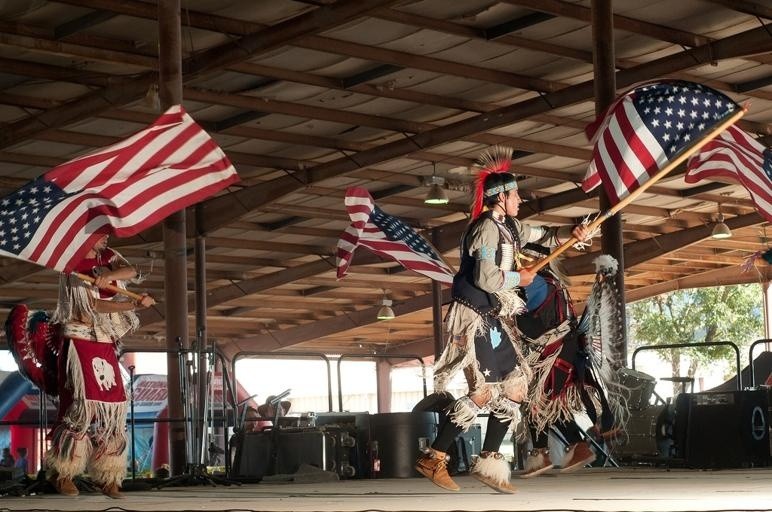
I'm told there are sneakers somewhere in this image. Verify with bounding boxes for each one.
[47,474,82,497]
[555,441,599,473]
[517,447,554,479]
[413,448,460,493]
[104,478,128,501]
[467,458,518,496]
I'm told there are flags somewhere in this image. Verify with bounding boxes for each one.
[683,119,771,225]
[0,99,242,276]
[332,182,456,287]
[580,75,743,209]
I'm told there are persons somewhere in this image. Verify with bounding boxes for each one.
[42,228,157,500]
[413,168,591,495]
[511,240,597,479]
[1,447,15,468]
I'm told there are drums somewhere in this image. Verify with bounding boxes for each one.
[617,369,654,411]
[619,404,669,461]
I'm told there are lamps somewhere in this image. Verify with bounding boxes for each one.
[712,201,733,239]
[376,288,395,319]
[424,161,449,204]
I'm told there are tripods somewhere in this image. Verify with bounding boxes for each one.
[151,354,243,488]
[20,387,56,494]
